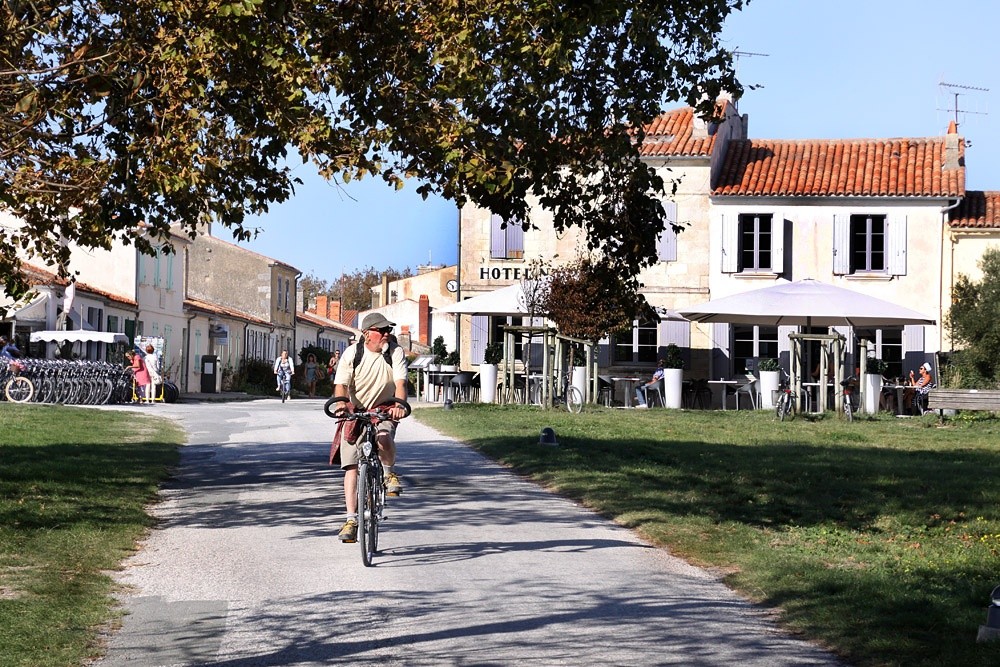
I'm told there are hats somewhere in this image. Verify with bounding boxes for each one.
[923,362,932,371]
[361,313,397,329]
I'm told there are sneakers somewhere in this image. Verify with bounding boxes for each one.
[337,520,359,540]
[381,472,403,493]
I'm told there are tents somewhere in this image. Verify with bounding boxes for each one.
[30,330,129,360]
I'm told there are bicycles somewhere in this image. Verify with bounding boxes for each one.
[536,371,583,414]
[324,396,411,567]
[276,371,291,403]
[775,368,799,422]
[0,358,179,405]
[840,375,856,423]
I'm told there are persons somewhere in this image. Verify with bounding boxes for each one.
[274,350,294,400]
[304,353,319,398]
[902,362,933,415]
[635,361,665,408]
[142,344,159,404]
[333,313,407,540]
[0,335,20,358]
[329,350,341,397]
[812,362,821,411]
[125,350,151,404]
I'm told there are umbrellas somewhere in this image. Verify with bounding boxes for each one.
[430,274,691,405]
[674,274,936,415]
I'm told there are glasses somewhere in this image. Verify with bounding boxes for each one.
[365,326,393,335]
[335,353,340,355]
[919,367,924,370]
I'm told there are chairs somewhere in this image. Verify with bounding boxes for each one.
[590,374,664,410]
[689,379,756,410]
[425,370,480,404]
[496,371,560,404]
[791,380,930,416]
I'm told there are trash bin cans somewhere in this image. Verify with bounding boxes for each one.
[201,355,220,392]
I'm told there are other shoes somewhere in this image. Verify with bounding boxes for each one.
[276,387,280,391]
[635,404,646,408]
[287,396,290,401]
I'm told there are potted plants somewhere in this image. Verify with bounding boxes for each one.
[865,355,888,415]
[567,348,586,404]
[441,351,459,402]
[427,335,447,402]
[479,342,503,403]
[664,342,686,410]
[758,358,780,410]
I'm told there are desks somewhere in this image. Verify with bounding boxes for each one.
[882,383,919,417]
[802,383,834,411]
[610,378,642,408]
[708,380,737,411]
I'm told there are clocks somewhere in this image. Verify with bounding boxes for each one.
[446,280,457,292]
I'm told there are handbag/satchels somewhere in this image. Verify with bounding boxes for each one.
[327,366,333,374]
[314,370,322,378]
